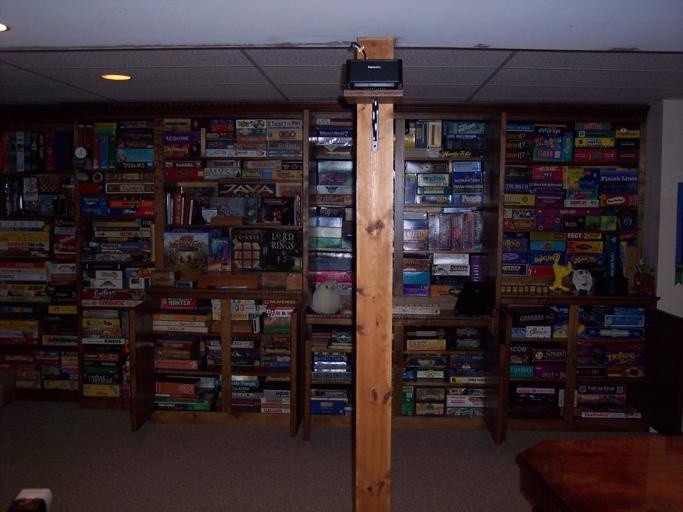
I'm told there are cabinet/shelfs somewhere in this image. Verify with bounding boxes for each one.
[0,112,646,439]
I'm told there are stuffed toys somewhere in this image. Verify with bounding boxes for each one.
[548,253,574,291]
[447,278,484,316]
[310,279,342,315]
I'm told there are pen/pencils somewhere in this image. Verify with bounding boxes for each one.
[632,257,655,276]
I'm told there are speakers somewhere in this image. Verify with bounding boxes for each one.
[346,56,404,90]
